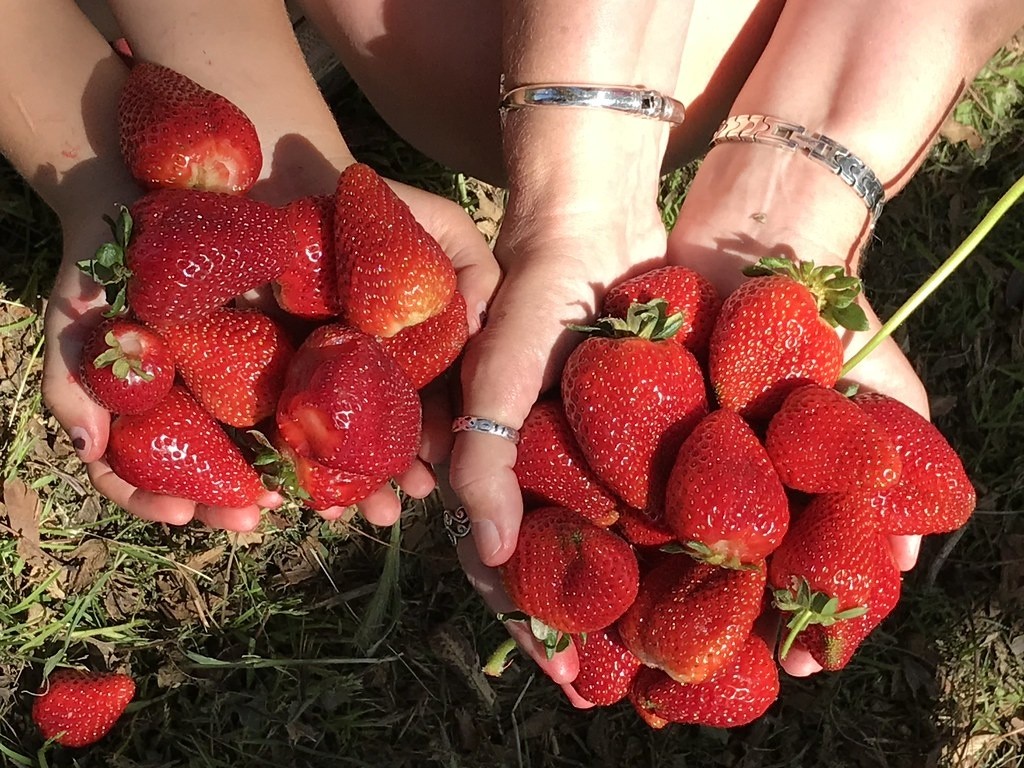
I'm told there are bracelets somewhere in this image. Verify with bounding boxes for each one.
[707,115,885,221]
[498,83,686,129]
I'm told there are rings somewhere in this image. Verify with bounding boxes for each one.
[443,505,472,546]
[452,416,520,445]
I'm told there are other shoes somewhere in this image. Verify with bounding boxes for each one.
[283,0,340,83]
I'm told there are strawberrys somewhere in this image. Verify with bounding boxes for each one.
[116,61,263,195]
[77,162,469,512]
[500,257,977,730]
[32,669,136,747]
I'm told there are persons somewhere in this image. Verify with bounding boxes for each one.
[297,0,1024,730]
[0,0,503,533]
